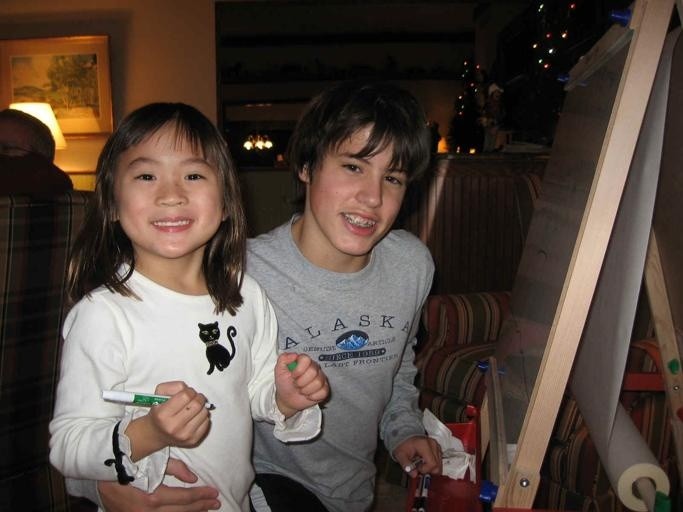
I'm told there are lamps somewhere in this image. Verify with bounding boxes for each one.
[243,134,273,150]
[8,102,66,151]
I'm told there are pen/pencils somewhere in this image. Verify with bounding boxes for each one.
[406,460,425,472]
[412,473,430,512]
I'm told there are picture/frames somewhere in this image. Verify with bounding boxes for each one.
[0,35,114,136]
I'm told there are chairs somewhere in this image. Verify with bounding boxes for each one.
[384,169,682,511]
[0,190,99,511]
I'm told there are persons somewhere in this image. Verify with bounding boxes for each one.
[62,86,443,512]
[46,102,330,511]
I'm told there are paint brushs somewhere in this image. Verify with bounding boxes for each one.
[99,388,216,414]
